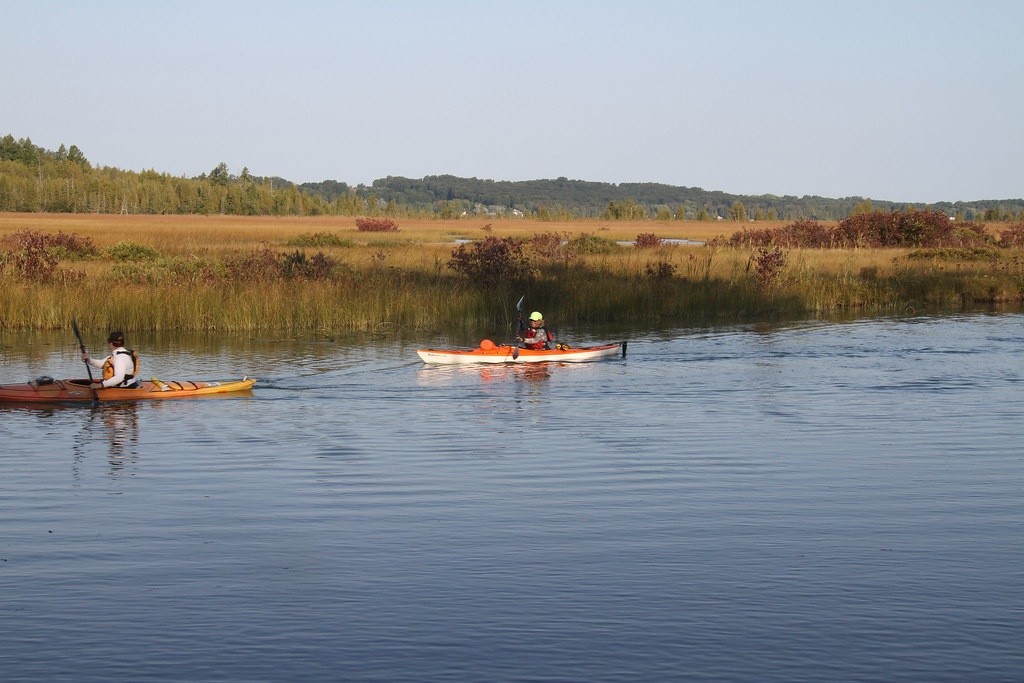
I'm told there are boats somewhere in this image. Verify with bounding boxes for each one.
[416,341,627,364]
[0,375,256,402]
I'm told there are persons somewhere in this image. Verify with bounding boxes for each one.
[515,312,548,351]
[81,331,139,389]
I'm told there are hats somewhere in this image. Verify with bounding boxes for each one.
[106,332,125,342]
[529,311,543,321]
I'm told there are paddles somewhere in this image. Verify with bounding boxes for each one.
[71,317,103,407]
[512,295,525,360]
